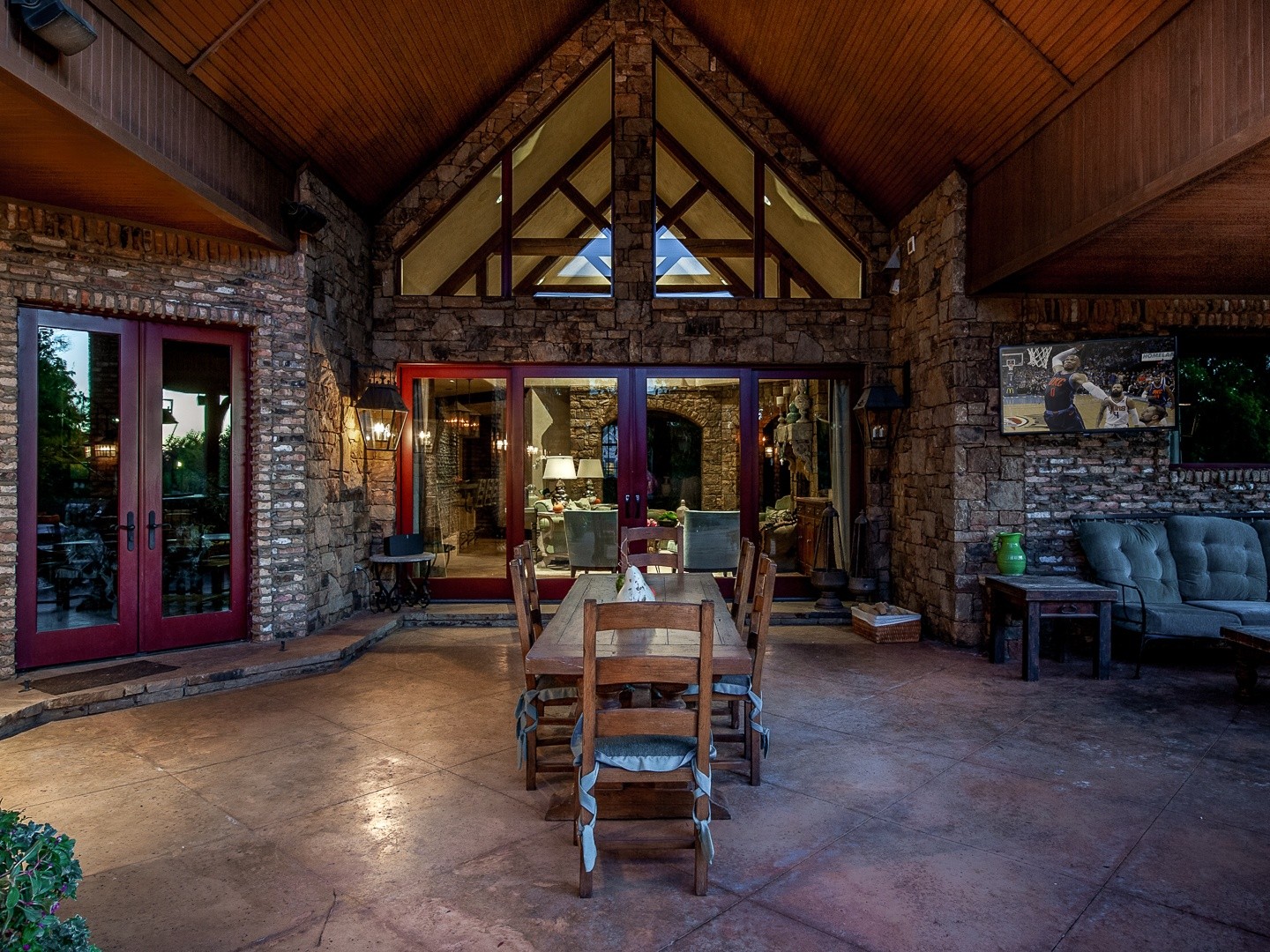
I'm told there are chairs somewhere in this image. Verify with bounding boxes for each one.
[758,492,794,573]
[507,560,582,792]
[619,524,684,573]
[515,541,548,640]
[649,536,754,727]
[562,509,617,577]
[571,596,719,897]
[534,500,571,567]
[682,509,740,577]
[684,553,778,787]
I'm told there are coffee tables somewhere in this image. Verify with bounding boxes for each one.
[1219,624,1270,719]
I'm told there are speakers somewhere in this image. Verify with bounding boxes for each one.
[384,533,423,556]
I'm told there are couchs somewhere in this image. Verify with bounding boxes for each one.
[1074,512,1270,680]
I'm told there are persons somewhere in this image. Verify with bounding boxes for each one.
[1141,373,1176,411]
[647,471,659,506]
[1139,404,1167,427]
[1043,346,1118,431]
[1095,383,1140,428]
[1002,361,1175,397]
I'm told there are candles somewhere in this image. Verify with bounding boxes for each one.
[776,396,784,405]
[782,386,791,394]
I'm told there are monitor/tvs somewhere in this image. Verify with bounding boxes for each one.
[999,333,1179,433]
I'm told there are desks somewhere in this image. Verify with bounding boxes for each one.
[525,572,754,822]
[368,551,433,611]
[590,503,618,509]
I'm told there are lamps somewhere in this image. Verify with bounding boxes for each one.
[353,367,412,451]
[576,457,604,504]
[441,378,468,435]
[464,379,485,438]
[413,405,442,455]
[852,363,912,452]
[542,452,577,508]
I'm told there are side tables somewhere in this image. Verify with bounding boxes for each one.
[987,575,1118,681]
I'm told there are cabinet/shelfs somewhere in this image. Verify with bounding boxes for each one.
[796,495,828,576]
